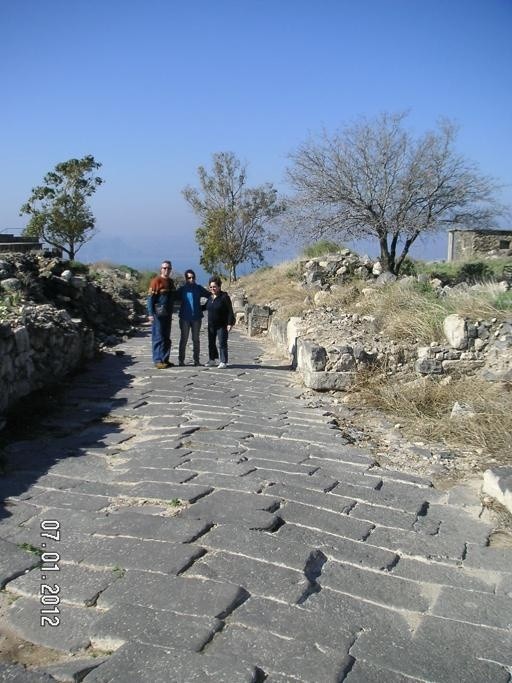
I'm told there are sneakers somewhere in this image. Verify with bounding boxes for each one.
[205,360,215,366]
[179,362,184,365]
[155,362,173,369]
[194,363,203,366]
[218,363,226,368]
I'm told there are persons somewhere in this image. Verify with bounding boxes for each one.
[147,260,176,368]
[200,275,235,369]
[175,268,211,365]
